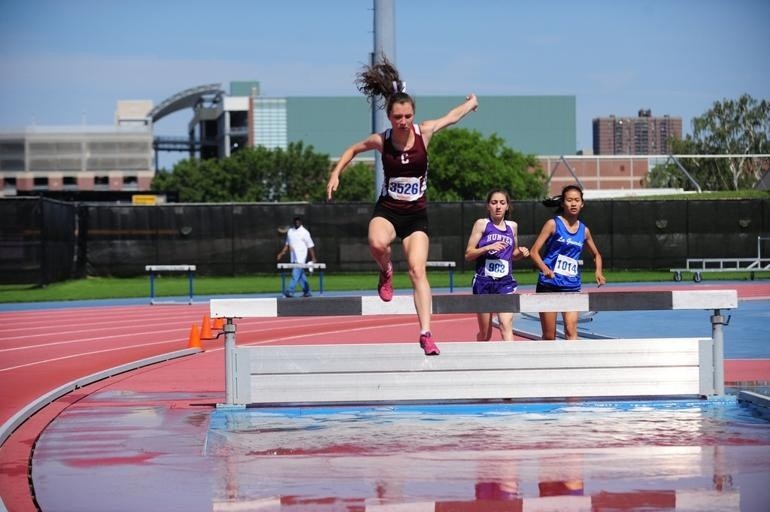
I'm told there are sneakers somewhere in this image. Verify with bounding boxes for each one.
[285,290,312,297]
[378,270,393,301]
[420,332,440,355]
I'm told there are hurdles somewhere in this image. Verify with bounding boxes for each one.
[209,290,738,408]
[425,260,457,292]
[276,262,327,296]
[146,265,195,305]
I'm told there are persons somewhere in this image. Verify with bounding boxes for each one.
[463,190,530,342]
[482,398,585,500]
[530,185,607,340]
[327,48,480,354]
[276,216,317,298]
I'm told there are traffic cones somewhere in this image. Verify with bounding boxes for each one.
[188,313,224,348]
